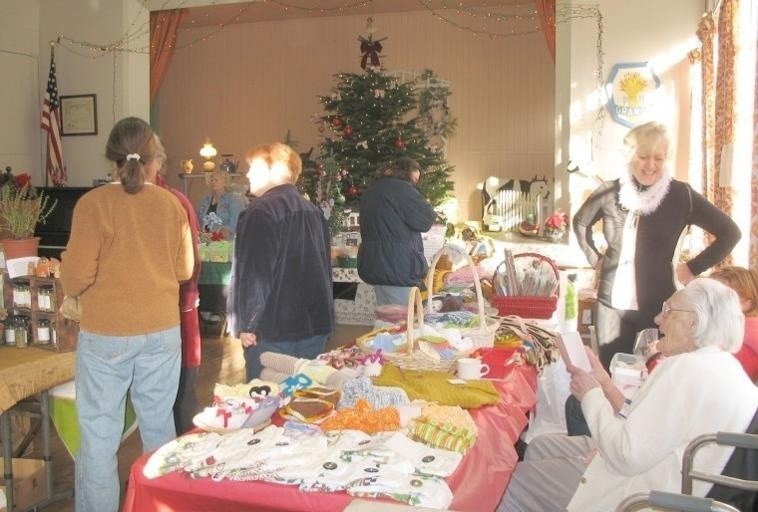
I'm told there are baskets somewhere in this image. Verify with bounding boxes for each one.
[491,252,562,320]
[354,285,456,375]
[425,243,500,350]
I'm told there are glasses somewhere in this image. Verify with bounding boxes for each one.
[661,300,693,316]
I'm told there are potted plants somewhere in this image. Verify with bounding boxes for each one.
[544,210,568,240]
[1,180,58,259]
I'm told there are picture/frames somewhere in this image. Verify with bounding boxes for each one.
[60,94,98,136]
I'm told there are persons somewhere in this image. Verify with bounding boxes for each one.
[56,116,194,512]
[145,132,202,438]
[357,156,437,309]
[571,120,741,378]
[492,276,758,512]
[195,169,247,239]
[564,264,756,437]
[224,143,337,384]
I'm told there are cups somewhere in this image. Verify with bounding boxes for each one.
[457,359,490,381]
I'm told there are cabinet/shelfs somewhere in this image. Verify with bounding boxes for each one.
[0,274,80,352]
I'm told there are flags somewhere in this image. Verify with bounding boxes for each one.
[38,58,69,189]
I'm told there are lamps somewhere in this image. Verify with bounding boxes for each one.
[199,143,217,171]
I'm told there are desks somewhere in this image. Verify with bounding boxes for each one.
[0,344,77,511]
[123,327,537,512]
[33,186,96,249]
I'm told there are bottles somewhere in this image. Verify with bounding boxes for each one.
[37,321,50,343]
[3,322,16,347]
[16,323,28,349]
[38,288,55,312]
[14,284,30,309]
[52,324,58,348]
